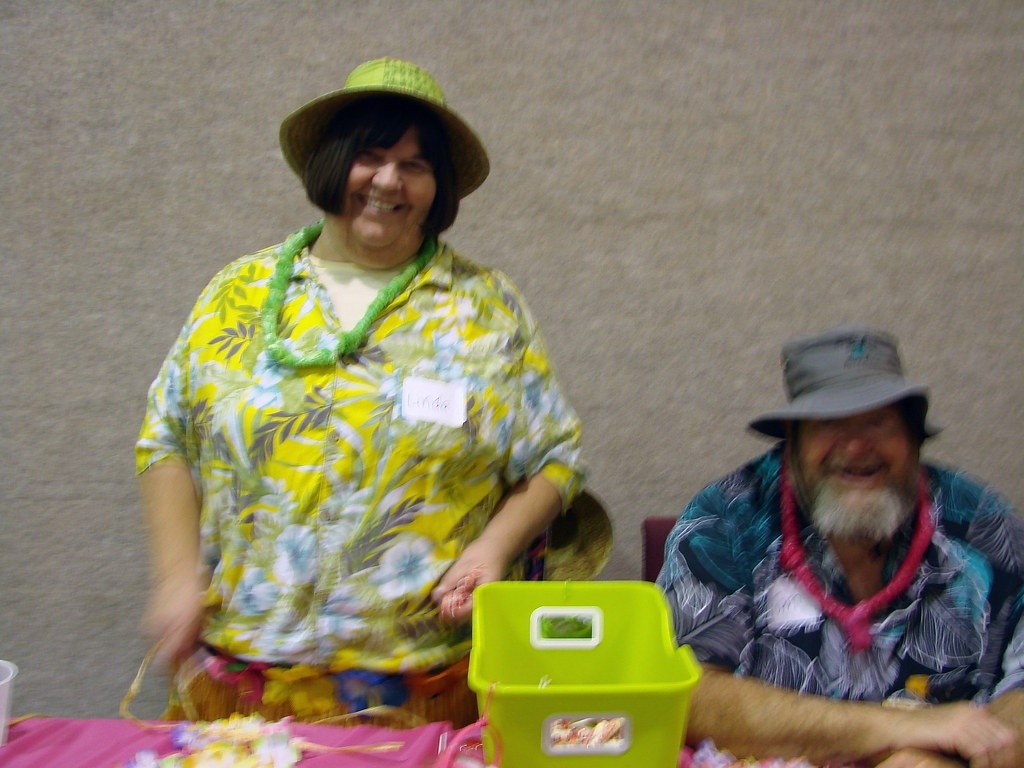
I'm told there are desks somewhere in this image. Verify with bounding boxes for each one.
[1,718,489,768]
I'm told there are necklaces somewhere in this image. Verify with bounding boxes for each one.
[780,462,932,651]
[263,222,437,368]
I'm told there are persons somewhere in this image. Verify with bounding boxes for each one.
[132,58,584,727]
[655,326,1024,768]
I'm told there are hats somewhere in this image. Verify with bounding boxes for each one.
[278,55,491,203]
[745,324,951,443]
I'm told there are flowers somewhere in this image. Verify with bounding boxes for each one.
[695,738,812,768]
[118,712,303,767]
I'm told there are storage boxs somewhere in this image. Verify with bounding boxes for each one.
[467,582,704,768]
